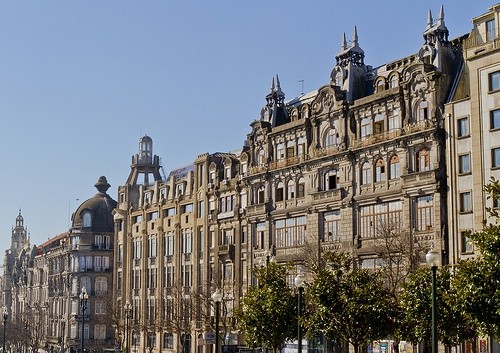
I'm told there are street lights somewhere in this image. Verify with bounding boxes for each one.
[123,300,133,353]
[425,247,441,353]
[2,309,8,353]
[79,286,89,353]
[294,274,306,353]
[212,289,221,353]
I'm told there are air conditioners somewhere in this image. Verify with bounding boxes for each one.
[239,175,243,180]
[208,182,213,189]
[240,208,244,213]
[223,180,228,184]
[207,215,213,219]
